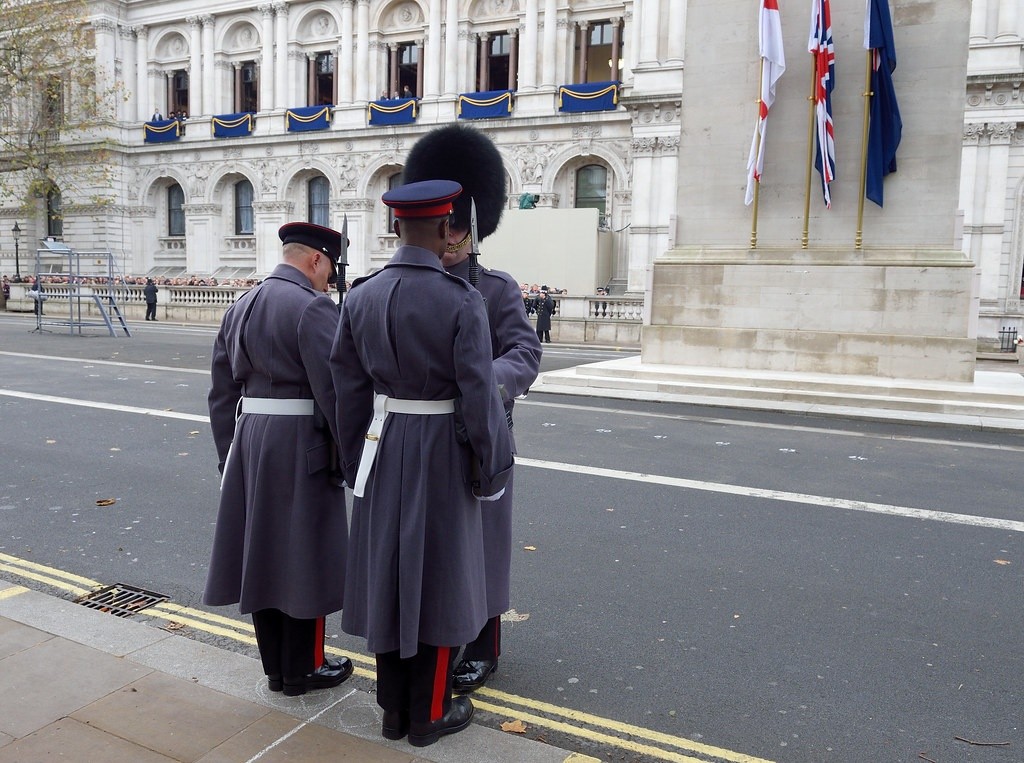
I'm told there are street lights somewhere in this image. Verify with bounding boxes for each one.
[11,220,24,283]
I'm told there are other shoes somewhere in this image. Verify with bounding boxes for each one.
[546,340,550,343]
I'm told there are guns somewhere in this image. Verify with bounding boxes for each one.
[467,197,488,306]
[335,213,349,315]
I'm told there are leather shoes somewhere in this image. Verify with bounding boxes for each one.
[282,656,354,696]
[382,709,408,740]
[451,657,498,691]
[268,674,283,692]
[408,696,475,747]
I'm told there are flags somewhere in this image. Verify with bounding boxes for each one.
[744,1,787,206]
[862,1,904,207]
[807,1,837,208]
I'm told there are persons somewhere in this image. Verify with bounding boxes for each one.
[151,109,189,135]
[380,84,413,101]
[2,273,353,305]
[330,125,542,747]
[203,221,356,696]
[144,279,158,321]
[519,284,610,343]
[32,278,47,316]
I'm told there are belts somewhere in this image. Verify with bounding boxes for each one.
[242,396,314,416]
[353,390,455,498]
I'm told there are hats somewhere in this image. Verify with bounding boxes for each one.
[597,287,604,292]
[382,180,463,220]
[278,222,350,284]
[540,285,548,294]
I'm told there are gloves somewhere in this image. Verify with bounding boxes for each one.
[472,486,506,501]
